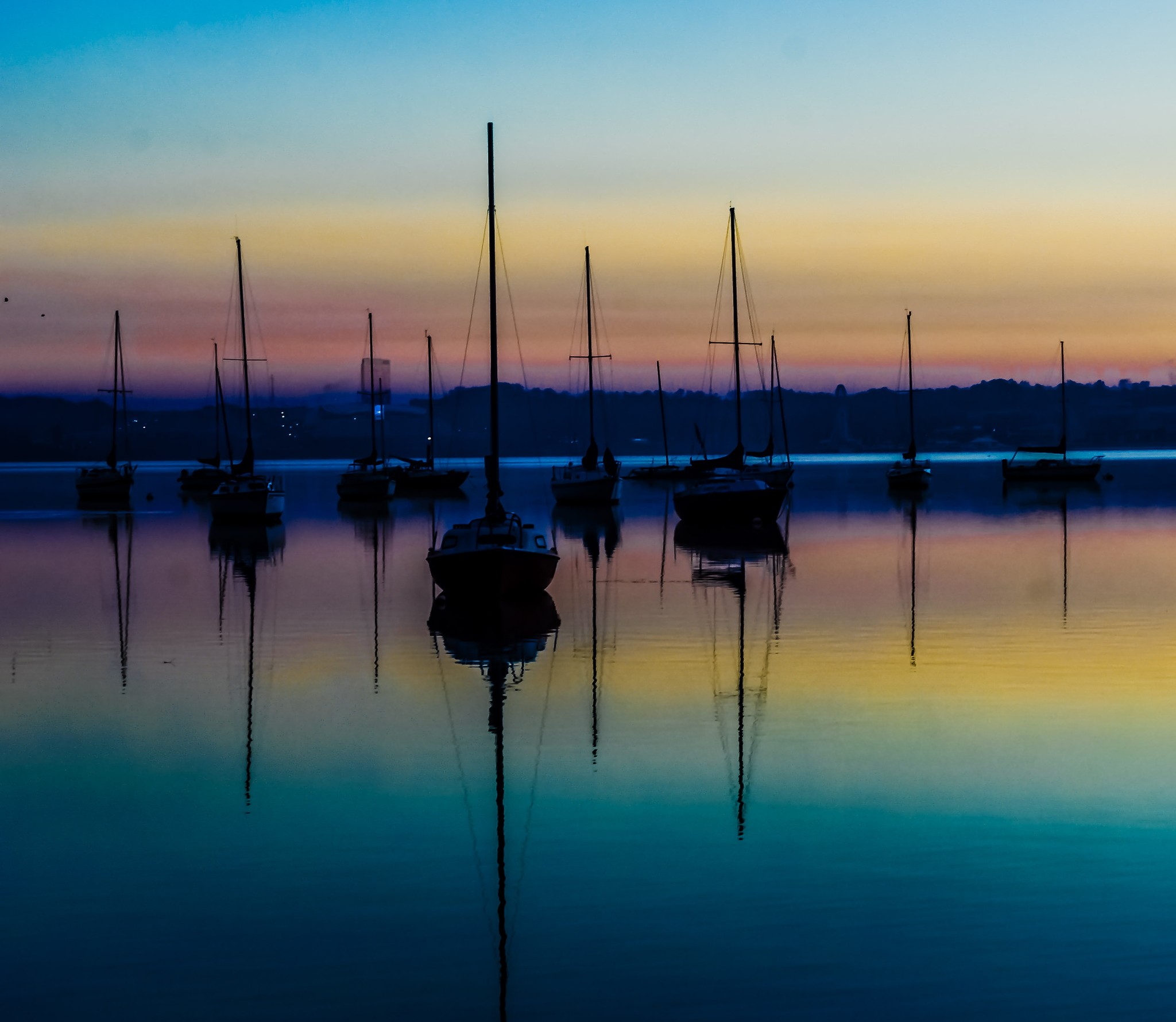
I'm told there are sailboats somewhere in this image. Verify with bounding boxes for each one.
[426,122,562,601]
[73,310,139,510]
[884,309,933,487]
[549,245,624,506]
[177,238,286,523]
[1001,342,1106,480]
[334,312,471,503]
[623,206,798,531]
